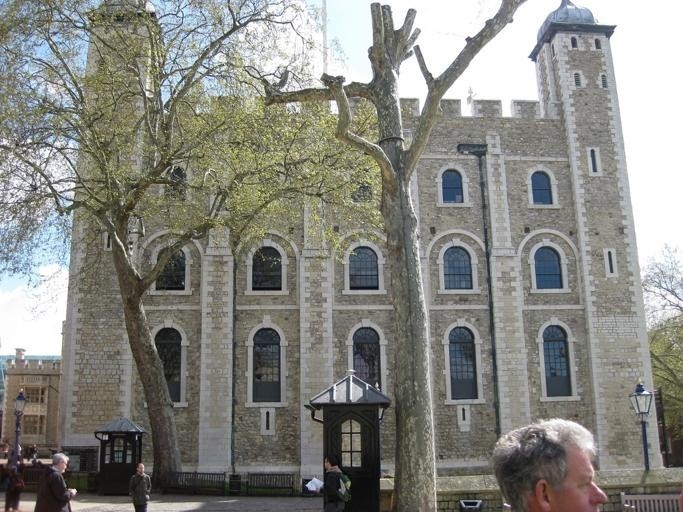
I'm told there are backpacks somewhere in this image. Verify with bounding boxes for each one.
[333,472,352,503]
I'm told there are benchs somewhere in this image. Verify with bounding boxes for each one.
[20,467,45,489]
[161,471,295,497]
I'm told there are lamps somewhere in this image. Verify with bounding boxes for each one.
[101,433,112,442]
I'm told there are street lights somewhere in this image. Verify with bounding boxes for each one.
[629,382,652,471]
[14,388,26,465]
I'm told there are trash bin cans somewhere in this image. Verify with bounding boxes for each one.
[459,499,482,512]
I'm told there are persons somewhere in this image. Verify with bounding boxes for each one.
[128,462,152,512]
[491,417,609,511]
[33,452,77,512]
[17,443,24,465]
[31,443,40,464]
[314,454,351,512]
[2,440,10,459]
[4,465,25,511]
